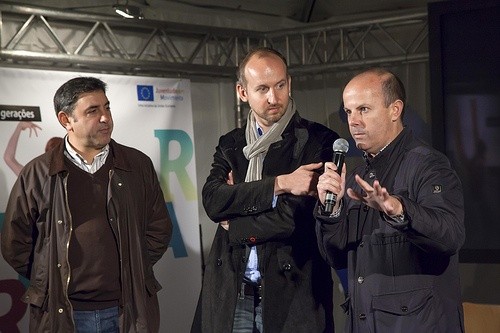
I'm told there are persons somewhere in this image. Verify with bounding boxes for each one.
[188,48,342,333]
[1,77,173,333]
[313,69,467,333]
[4,121,62,178]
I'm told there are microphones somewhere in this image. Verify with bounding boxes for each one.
[324,138,350,214]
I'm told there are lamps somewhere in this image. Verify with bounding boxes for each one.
[112,0,144,19]
